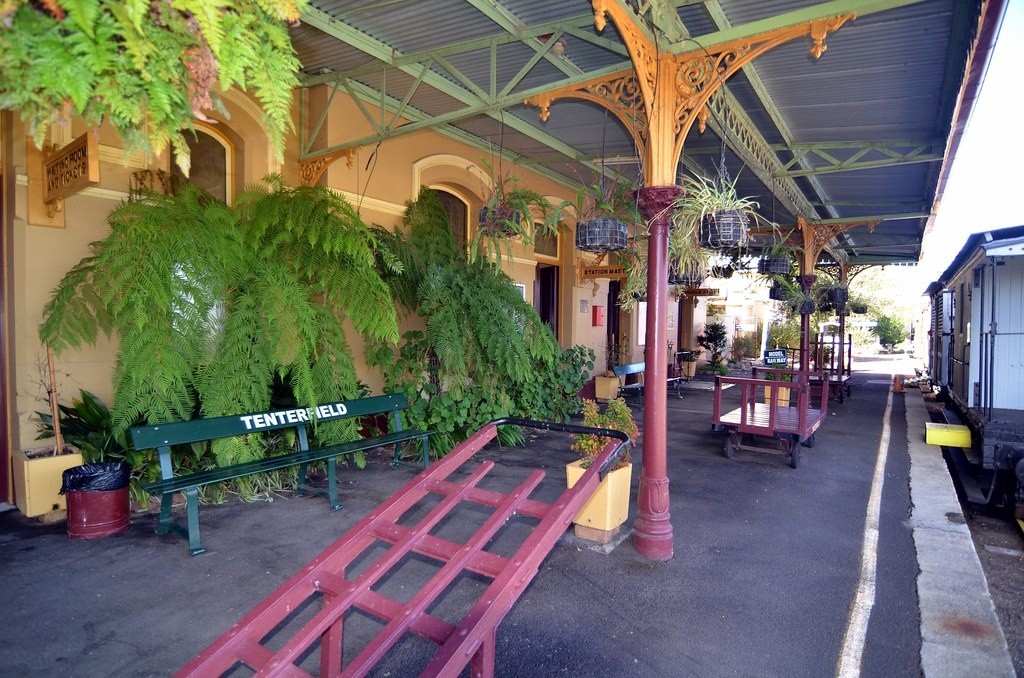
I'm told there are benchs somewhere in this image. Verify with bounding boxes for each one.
[612,362,685,411]
[130,393,438,557]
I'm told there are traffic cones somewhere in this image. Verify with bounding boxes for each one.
[890,376,902,393]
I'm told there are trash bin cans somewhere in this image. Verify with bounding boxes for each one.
[678,351,697,381]
[63,462,131,539]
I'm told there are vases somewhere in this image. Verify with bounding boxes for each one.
[14,442,82,518]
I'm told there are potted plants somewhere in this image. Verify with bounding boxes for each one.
[697,321,728,376]
[811,345,833,369]
[649,163,871,316]
[35,150,650,541]
[765,361,797,407]
[593,335,629,405]
